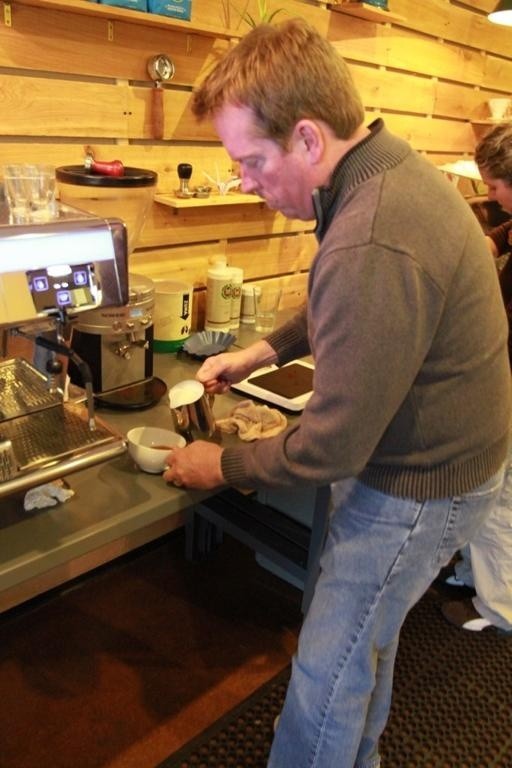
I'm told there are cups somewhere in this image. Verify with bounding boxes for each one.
[2,161,57,219]
[168,380,217,443]
[253,286,283,333]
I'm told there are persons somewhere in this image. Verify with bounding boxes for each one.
[412,116,511,639]
[158,13,512,767]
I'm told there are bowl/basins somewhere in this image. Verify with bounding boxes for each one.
[127,425,187,475]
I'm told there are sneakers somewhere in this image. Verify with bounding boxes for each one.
[441,574,478,635]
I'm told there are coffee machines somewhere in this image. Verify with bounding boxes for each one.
[64,272,167,409]
